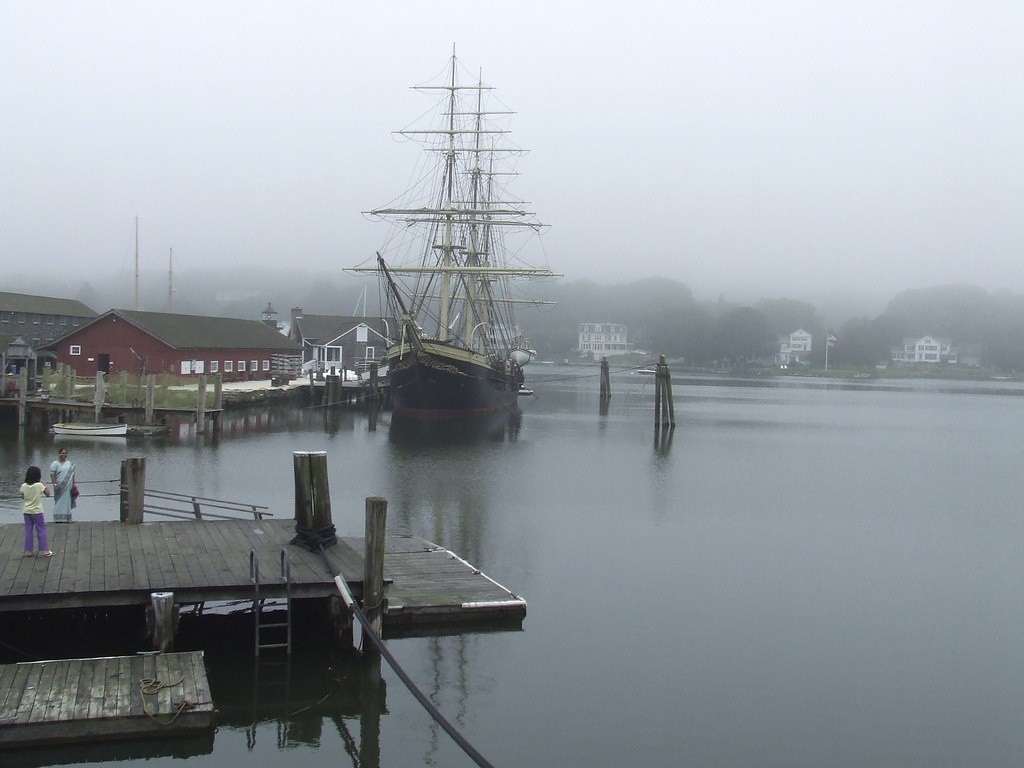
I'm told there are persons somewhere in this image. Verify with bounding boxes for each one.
[50,448,77,523]
[20,467,56,557]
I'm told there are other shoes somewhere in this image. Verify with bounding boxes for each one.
[44,551,56,557]
[23,552,32,557]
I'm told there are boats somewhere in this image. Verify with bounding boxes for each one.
[636,367,656,375]
[52,422,129,436]
[52,434,128,448]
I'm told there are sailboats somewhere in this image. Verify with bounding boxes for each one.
[340,41,564,421]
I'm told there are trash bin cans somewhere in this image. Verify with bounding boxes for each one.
[41,389,50,402]
[272,375,280,387]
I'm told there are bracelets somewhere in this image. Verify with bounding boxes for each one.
[54,484,57,486]
[73,483,77,485]
